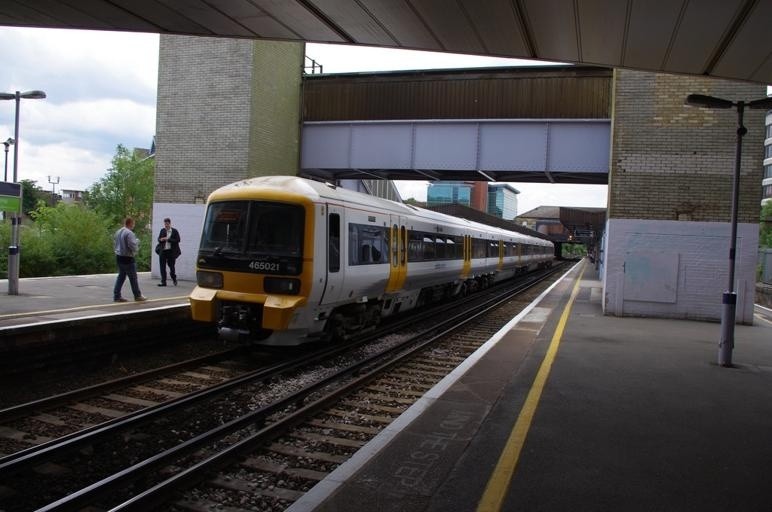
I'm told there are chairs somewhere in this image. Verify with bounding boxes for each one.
[135,296,146,301]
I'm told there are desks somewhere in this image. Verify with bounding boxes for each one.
[684,92,772,369]
[1,137,16,220]
[0,91,46,294]
[47,175,60,206]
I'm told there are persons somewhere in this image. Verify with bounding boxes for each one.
[112,217,148,301]
[155,218,180,287]
